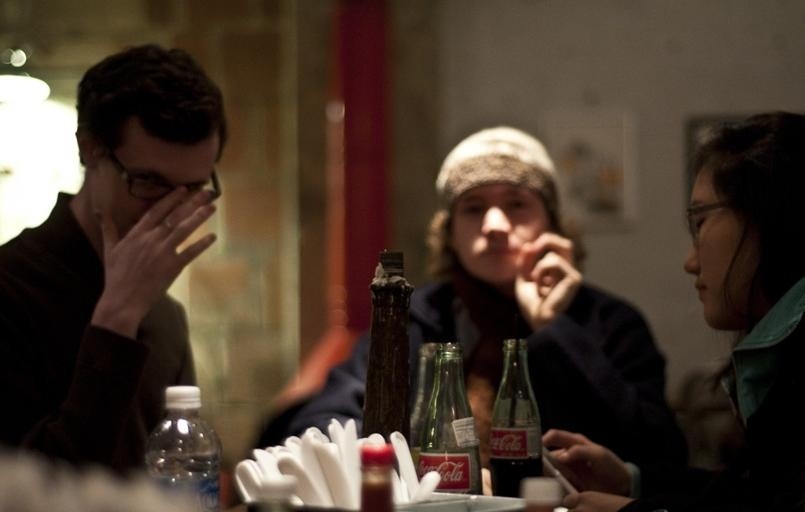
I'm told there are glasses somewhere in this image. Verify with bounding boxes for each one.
[685,200,728,250]
[104,150,221,204]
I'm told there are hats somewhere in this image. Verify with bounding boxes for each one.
[435,125,559,214]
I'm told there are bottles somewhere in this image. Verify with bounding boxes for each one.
[409,343,437,467]
[362,251,415,438]
[144,385,221,512]
[414,342,485,496]
[485,339,543,499]
[359,446,395,512]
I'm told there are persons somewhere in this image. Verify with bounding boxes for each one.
[0,45,231,512]
[291,125,690,465]
[541,112,805,512]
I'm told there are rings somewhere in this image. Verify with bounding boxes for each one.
[163,219,173,231]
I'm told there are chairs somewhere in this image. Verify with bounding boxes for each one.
[669,368,734,467]
